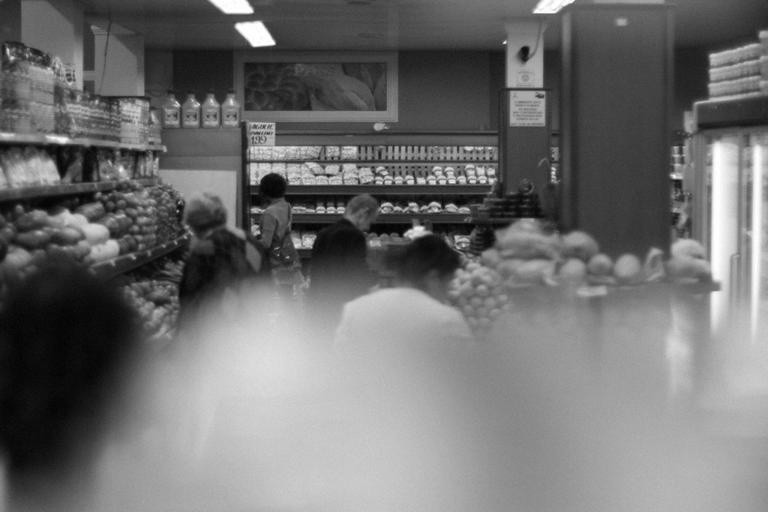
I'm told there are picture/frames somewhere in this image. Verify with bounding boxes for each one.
[232,49,399,123]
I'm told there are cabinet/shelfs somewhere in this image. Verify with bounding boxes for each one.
[3,132,193,318]
[236,122,504,261]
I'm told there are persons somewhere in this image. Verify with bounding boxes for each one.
[173,190,271,339]
[0,250,151,512]
[256,173,306,301]
[303,193,380,346]
[331,234,474,365]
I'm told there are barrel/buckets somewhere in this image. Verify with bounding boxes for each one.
[161,91,241,128]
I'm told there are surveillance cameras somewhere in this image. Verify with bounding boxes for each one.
[520,45,531,62]
